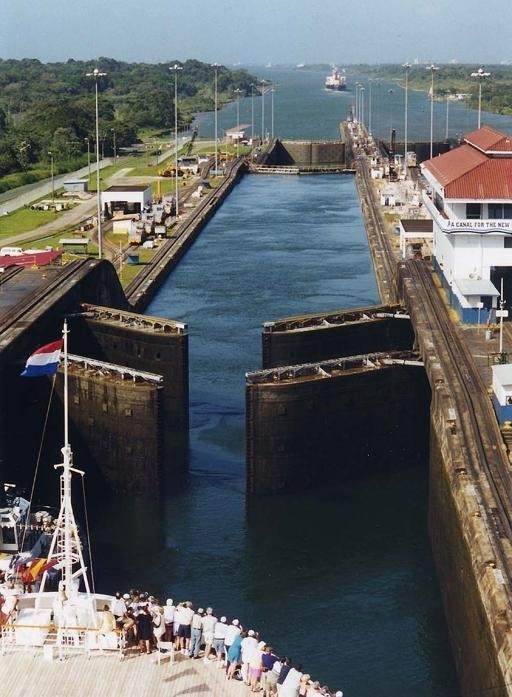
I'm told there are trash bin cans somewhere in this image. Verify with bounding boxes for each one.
[486,331,492,339]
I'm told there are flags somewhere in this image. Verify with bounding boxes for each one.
[20,560,61,594]
[18,339,65,379]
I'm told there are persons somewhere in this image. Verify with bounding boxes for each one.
[108,588,344,697]
[0,581,19,602]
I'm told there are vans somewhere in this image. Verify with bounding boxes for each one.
[1,246,25,256]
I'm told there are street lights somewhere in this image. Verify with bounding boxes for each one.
[168,62,186,218]
[469,69,491,131]
[259,77,267,141]
[110,127,118,165]
[250,81,256,139]
[354,80,366,131]
[48,150,56,204]
[85,67,110,259]
[424,64,439,158]
[233,86,242,161]
[401,61,410,165]
[84,136,92,185]
[269,86,276,140]
[209,60,223,180]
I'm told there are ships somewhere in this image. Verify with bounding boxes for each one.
[323,69,348,89]
